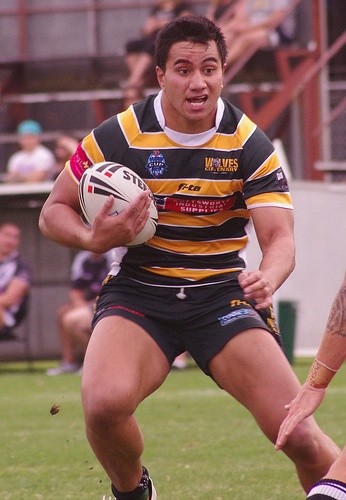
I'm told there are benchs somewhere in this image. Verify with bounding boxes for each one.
[0,0,311,373]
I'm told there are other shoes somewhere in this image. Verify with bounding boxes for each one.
[47,363,77,375]
[173,361,186,369]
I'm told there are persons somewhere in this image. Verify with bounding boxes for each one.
[274,273,346,500]
[0,119,57,183]
[52,135,84,181]
[119,0,302,88]
[0,219,33,340]
[46,246,186,377]
[38,14,342,500]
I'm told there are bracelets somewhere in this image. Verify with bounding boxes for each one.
[306,358,339,389]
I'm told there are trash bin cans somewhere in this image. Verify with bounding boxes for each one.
[277,299,297,365]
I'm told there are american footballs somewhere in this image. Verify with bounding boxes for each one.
[80,160,159,248]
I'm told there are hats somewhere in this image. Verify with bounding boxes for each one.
[18,120,42,135]
